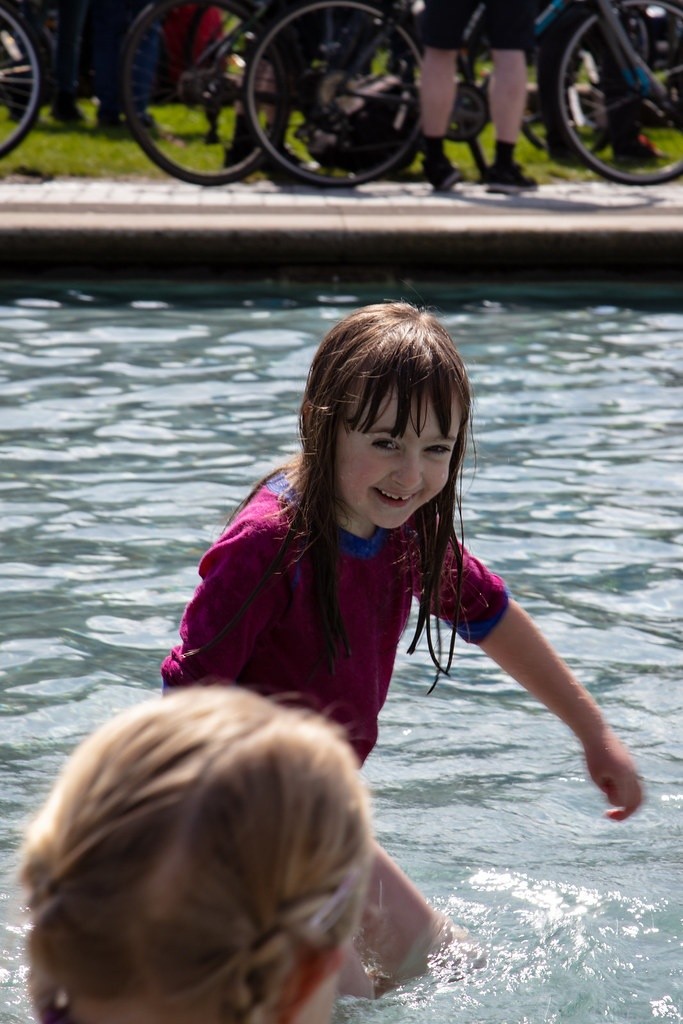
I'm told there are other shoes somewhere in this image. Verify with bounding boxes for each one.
[97,111,124,127]
[421,154,460,191]
[50,104,84,123]
[281,146,320,171]
[487,164,537,191]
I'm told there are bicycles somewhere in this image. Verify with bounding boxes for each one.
[0,0,682,198]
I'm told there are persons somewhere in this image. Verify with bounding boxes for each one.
[161,302,645,998]
[14,682,374,1024]
[0,1,683,191]
